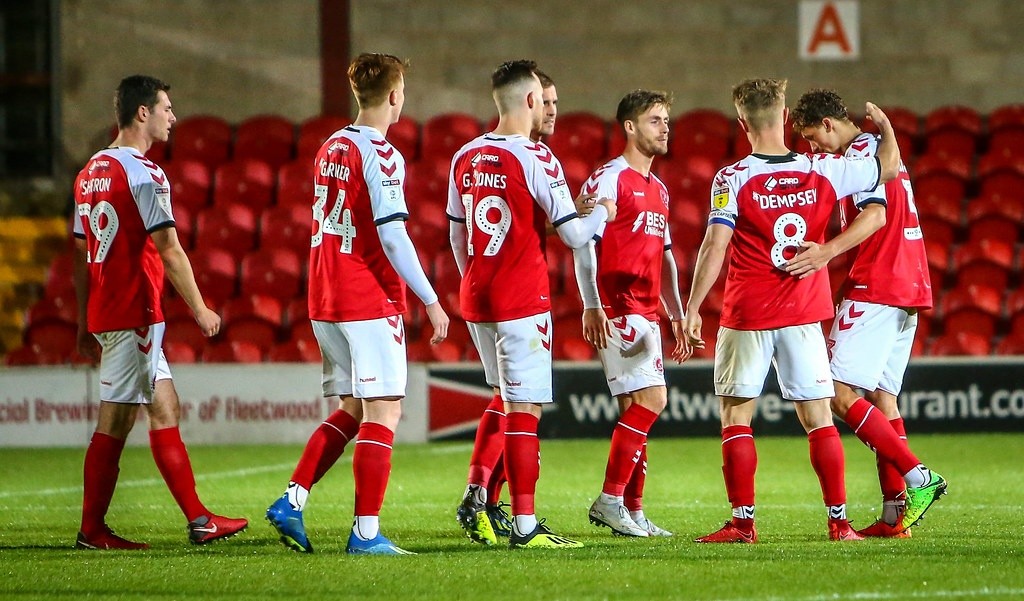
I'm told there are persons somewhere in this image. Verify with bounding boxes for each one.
[446,59,619,545]
[785,90,946,537]
[684,76,902,543]
[572,92,693,538]
[266,52,449,556]
[73,74,248,550]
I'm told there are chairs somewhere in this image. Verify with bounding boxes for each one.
[0,107,1024,362]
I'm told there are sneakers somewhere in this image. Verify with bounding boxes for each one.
[509,518,584,549]
[346,528,418,556]
[635,515,673,537]
[694,520,757,543]
[186,515,248,546]
[857,516,912,538]
[829,520,868,541]
[485,501,512,537]
[265,492,314,554]
[589,495,649,538]
[76,524,149,550]
[895,470,947,529]
[456,492,498,547]
